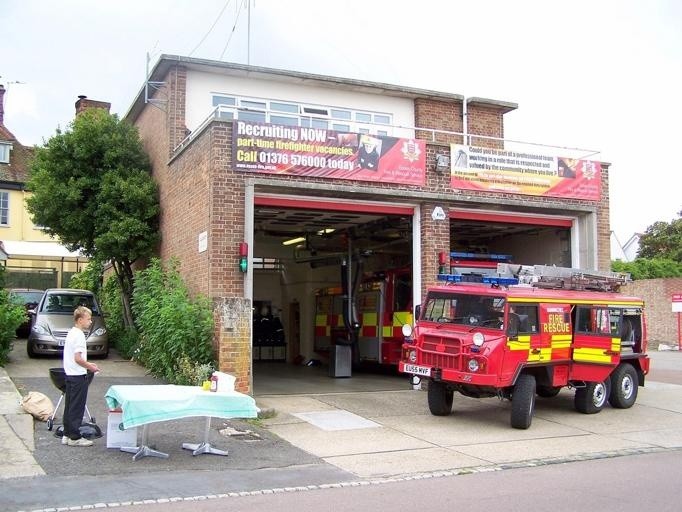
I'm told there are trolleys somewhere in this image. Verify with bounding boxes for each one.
[42,365,97,431]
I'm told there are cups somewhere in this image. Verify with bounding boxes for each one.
[202,380,210,391]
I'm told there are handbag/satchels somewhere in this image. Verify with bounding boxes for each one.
[19,391,57,421]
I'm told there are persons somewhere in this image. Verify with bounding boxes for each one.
[58,306,101,447]
[357,135,380,172]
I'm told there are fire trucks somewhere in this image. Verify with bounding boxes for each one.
[313,252,515,372]
[396,261,653,431]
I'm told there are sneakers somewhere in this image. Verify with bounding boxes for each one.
[68,437,93,447]
[61,436,68,445]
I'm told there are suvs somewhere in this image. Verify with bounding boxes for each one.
[26,287,111,359]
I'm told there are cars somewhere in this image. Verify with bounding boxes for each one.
[5,286,46,331]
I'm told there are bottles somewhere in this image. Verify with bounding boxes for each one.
[209,372,218,392]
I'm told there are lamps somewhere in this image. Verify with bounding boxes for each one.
[435,154,449,172]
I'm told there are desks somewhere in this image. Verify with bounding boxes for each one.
[111,385,252,462]
